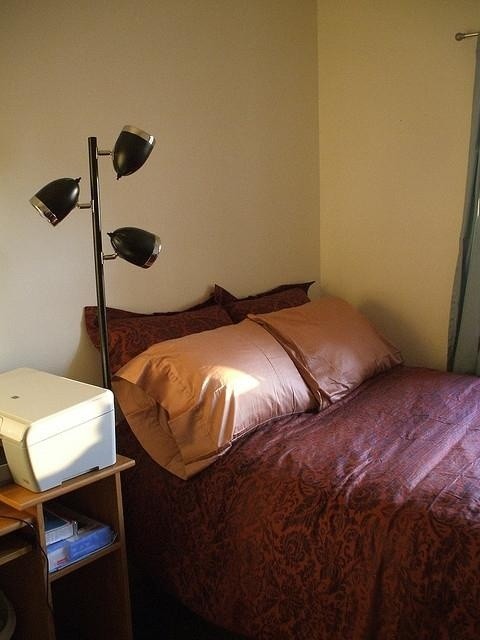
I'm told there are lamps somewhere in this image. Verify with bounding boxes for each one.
[28,124,163,388]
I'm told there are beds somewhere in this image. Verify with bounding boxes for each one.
[116,365,480,640]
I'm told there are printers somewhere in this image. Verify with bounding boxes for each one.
[0,366,117,494]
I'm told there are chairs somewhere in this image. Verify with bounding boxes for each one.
[214,279,316,319]
[113,316,318,480]
[247,290,405,413]
[82,297,241,371]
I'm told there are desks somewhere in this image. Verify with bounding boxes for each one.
[0,453,136,640]
